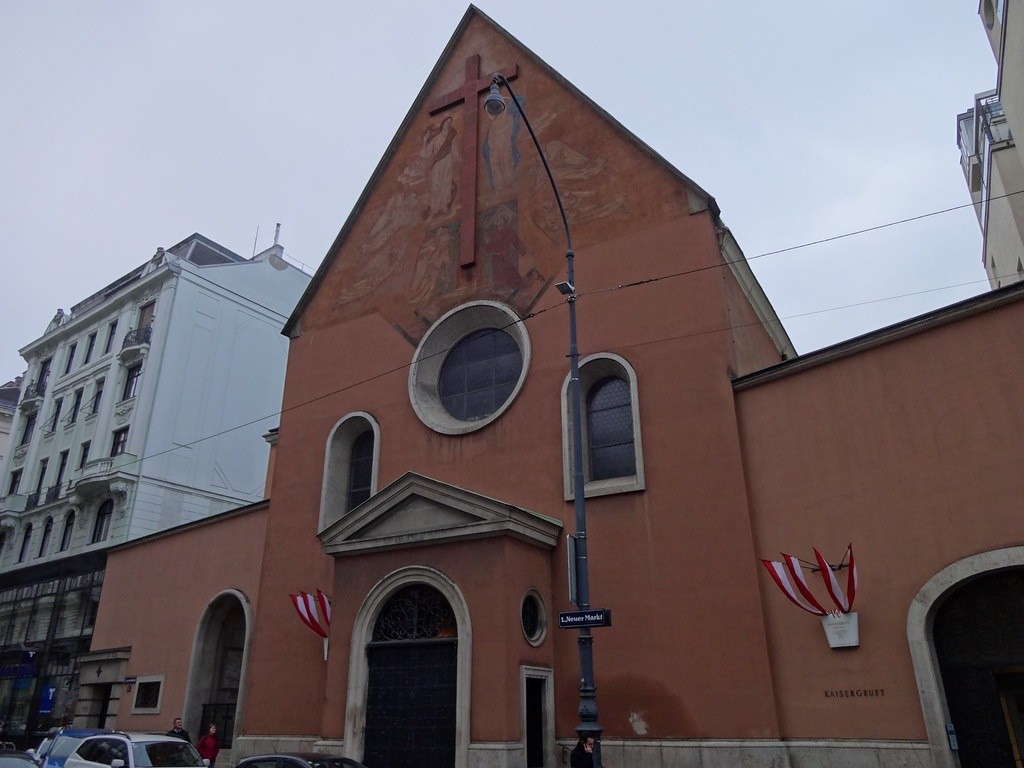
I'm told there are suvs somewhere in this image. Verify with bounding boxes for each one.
[64,732,210,767]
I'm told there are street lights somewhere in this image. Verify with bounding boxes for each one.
[483,71,604,767]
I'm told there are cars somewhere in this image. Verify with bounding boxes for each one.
[235,750,366,768]
[0,726,117,768]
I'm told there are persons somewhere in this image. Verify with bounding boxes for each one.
[166,718,191,744]
[570,734,594,768]
[197,724,220,768]
